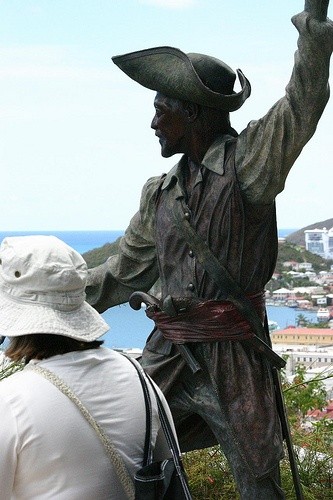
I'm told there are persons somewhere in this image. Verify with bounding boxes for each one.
[0,234,183,500]
[85,0,333,500]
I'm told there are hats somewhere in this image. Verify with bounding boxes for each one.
[0,236,110,341]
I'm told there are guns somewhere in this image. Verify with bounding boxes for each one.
[127,292,202,377]
[162,294,287,372]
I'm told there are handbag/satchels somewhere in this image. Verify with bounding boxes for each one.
[114,351,193,500]
